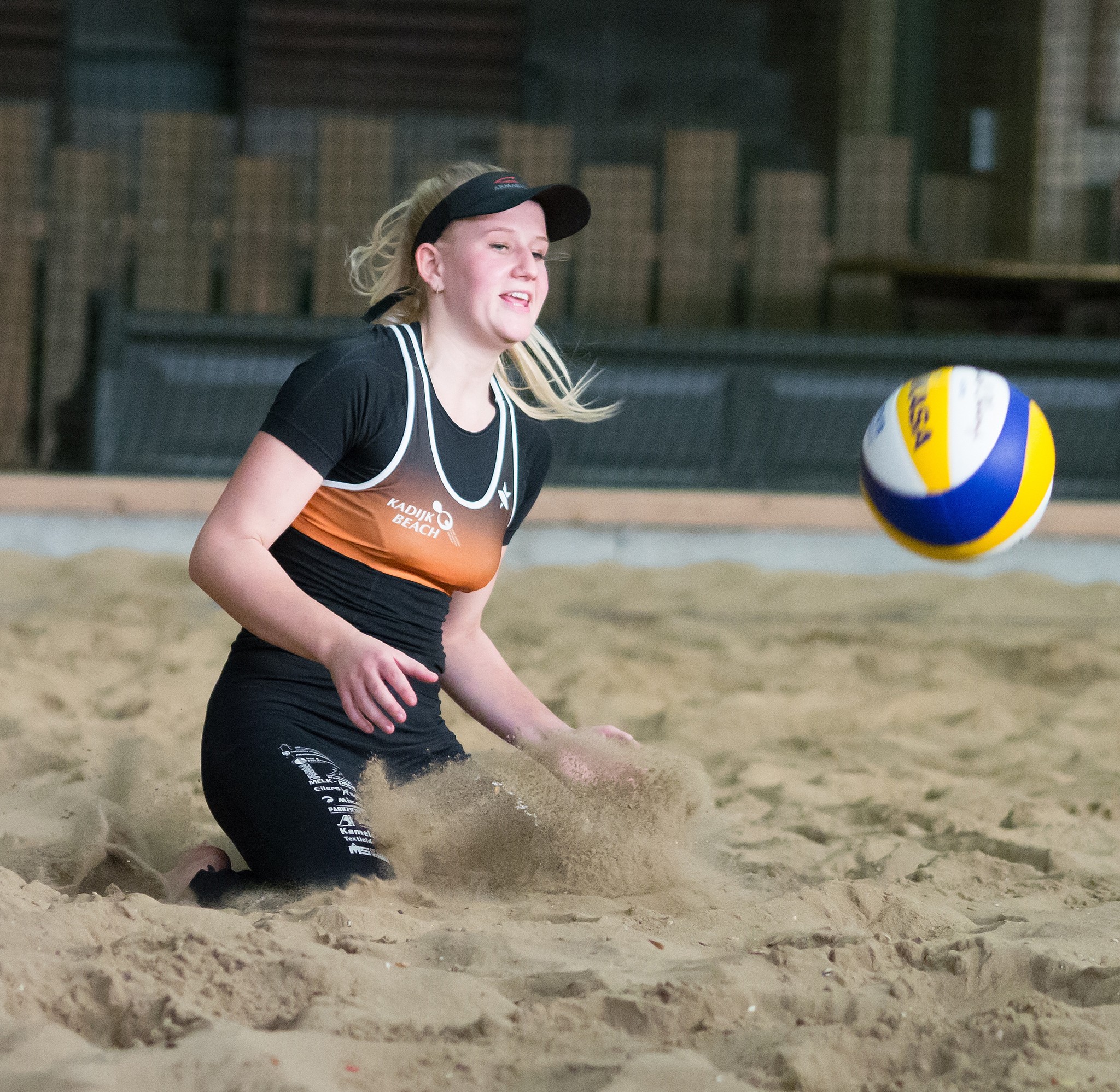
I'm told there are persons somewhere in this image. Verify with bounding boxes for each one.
[163,159,645,916]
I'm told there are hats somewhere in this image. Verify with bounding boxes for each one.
[360,171,592,324]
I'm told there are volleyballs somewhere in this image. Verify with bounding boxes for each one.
[861,366,1057,562]
